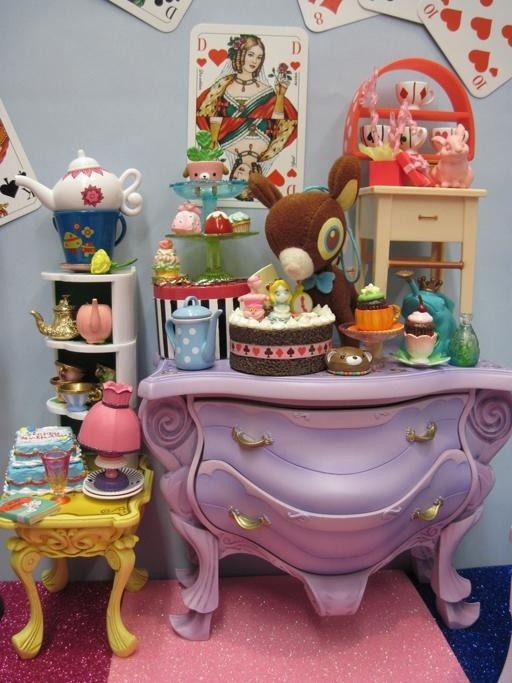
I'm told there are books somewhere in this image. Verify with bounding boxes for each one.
[0,491,60,526]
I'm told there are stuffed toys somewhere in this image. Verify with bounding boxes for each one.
[246,151,362,350]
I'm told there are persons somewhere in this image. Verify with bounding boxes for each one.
[195,116,297,201]
[195,32,297,118]
